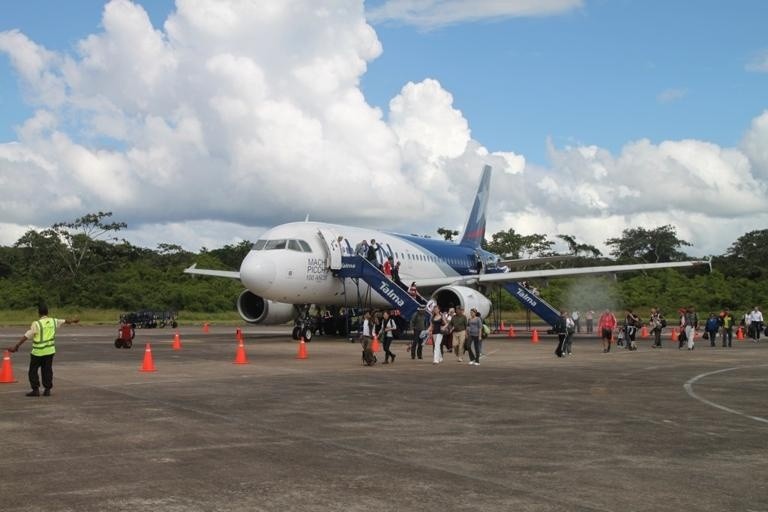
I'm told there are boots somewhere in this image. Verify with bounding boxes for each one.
[390,354,396,363]
[381,357,389,364]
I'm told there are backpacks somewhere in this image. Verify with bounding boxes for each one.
[651,314,668,328]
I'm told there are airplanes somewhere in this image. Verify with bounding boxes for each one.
[175,161,719,345]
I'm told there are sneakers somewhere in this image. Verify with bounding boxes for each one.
[26,389,40,397]
[555,330,761,357]
[468,360,481,366]
[432,358,444,364]
[43,389,51,396]
[457,357,463,362]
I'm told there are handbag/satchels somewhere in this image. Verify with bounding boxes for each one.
[441,325,450,334]
[390,318,401,339]
[633,314,643,329]
[425,337,433,345]
[377,331,386,343]
[482,324,491,340]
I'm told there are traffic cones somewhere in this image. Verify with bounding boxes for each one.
[735,325,744,341]
[500,320,504,331]
[202,321,208,332]
[530,327,540,344]
[229,337,249,365]
[135,341,156,372]
[669,328,676,342]
[507,323,517,337]
[0,347,19,383]
[296,334,309,359]
[233,326,242,339]
[640,325,649,337]
[171,329,182,350]
[370,333,381,352]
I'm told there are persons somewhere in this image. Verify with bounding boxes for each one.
[358,238,483,367]
[8,304,81,397]
[552,305,764,358]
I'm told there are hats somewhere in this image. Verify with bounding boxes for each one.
[416,304,428,311]
